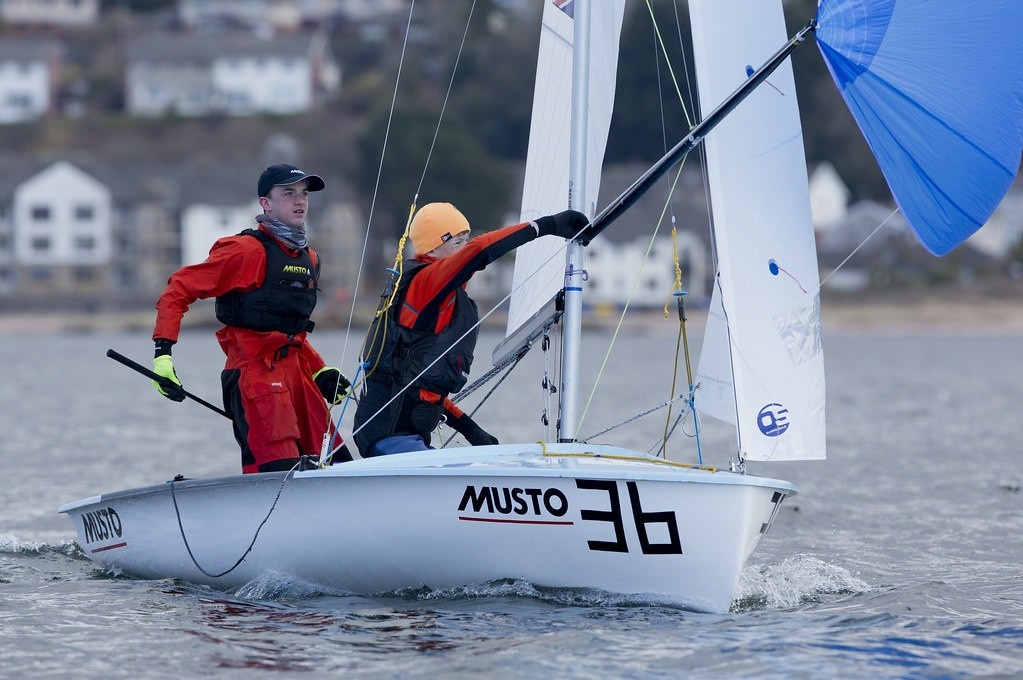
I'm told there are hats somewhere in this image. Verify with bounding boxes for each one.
[409,202,471,252]
[257,164,325,197]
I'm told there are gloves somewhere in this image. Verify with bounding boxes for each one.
[534,210,592,241]
[312,367,351,405]
[467,428,499,446]
[152,355,186,402]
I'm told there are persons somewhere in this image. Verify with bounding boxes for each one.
[353,202,592,460]
[151,164,354,475]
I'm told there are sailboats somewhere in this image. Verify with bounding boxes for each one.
[57,0,828,618]
